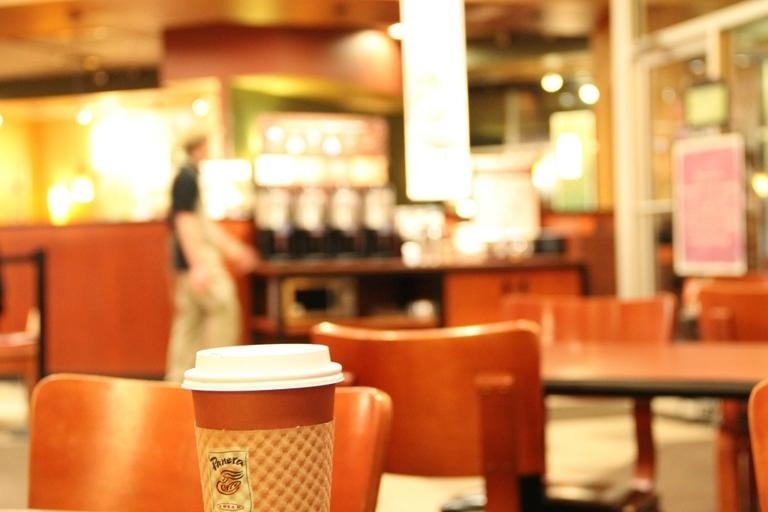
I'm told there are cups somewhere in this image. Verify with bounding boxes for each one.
[179,341,345,512]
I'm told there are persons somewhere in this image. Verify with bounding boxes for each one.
[163,121,258,386]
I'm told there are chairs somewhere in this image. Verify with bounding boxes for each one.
[29,371,393,512]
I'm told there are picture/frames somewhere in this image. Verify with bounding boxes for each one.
[672,133,747,278]
